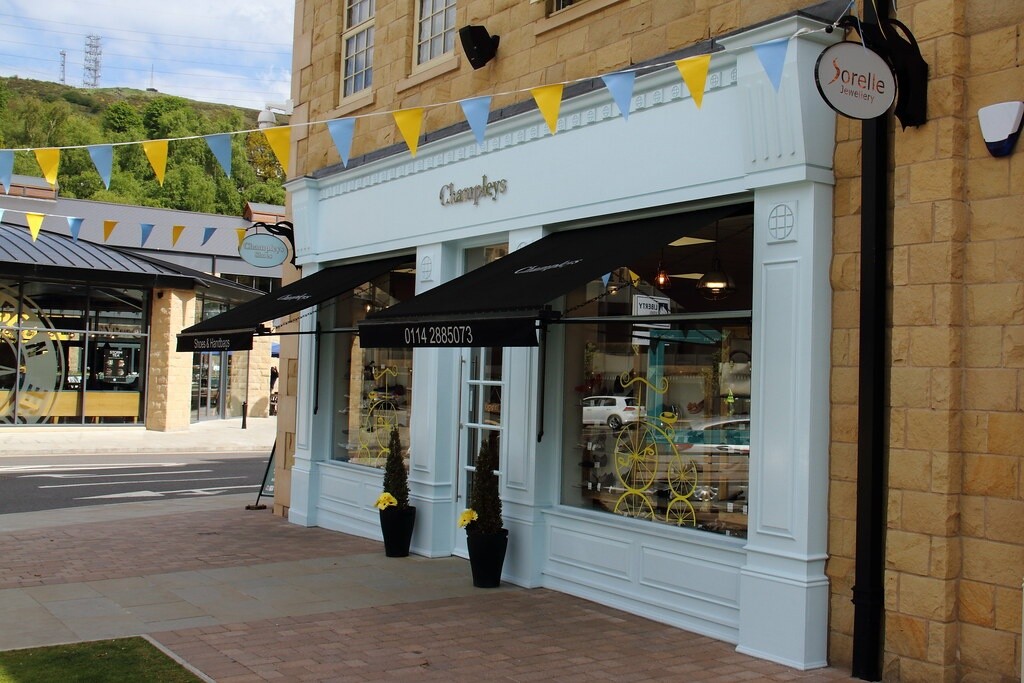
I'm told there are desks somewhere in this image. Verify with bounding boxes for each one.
[0,389,140,424]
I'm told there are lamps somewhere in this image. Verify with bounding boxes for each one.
[654,246,672,289]
[696,220,736,302]
[459,25,501,70]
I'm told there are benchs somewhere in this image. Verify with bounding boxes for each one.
[628,454,749,522]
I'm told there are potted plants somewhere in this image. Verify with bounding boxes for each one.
[464,438,509,588]
[378,427,417,558]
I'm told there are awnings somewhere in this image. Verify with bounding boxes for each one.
[176,252,413,352]
[358,203,707,350]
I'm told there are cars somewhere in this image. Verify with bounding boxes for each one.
[370,387,401,409]
[582,395,647,432]
[668,419,750,459]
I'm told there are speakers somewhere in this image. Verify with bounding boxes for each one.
[458,25,497,70]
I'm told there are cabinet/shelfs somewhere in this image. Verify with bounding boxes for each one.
[338,367,411,454]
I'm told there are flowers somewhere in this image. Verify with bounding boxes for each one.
[457,508,479,527]
[375,492,398,511]
